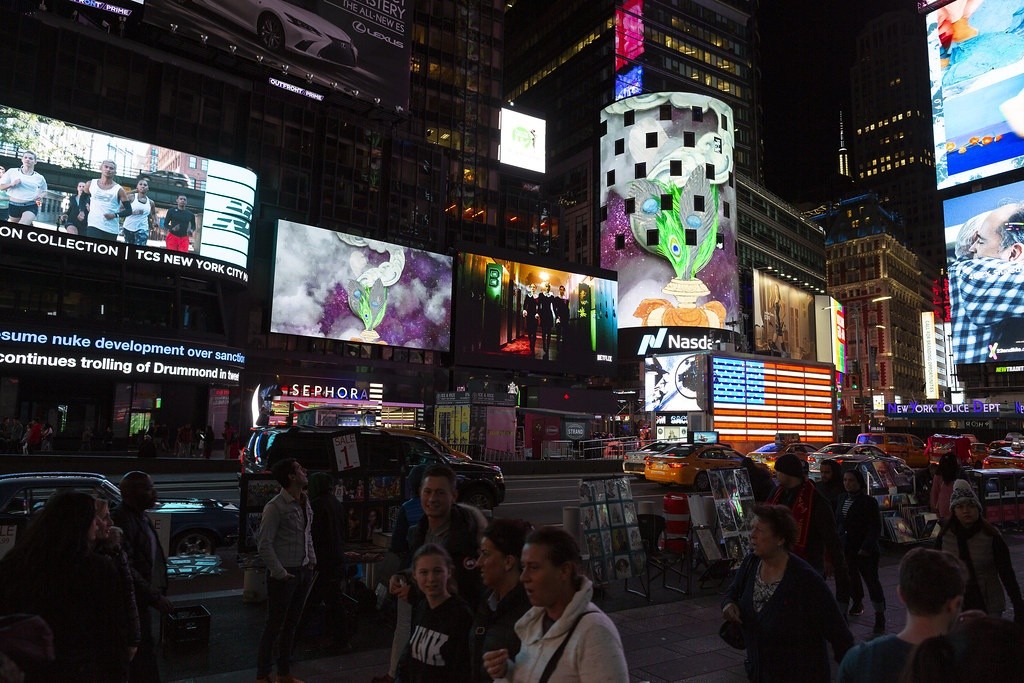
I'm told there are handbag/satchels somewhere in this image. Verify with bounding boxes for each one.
[719,553,756,651]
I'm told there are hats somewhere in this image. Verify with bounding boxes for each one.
[774,454,803,477]
[948,479,982,511]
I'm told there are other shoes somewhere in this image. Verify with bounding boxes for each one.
[276,672,306,683]
[872,611,887,636]
[849,601,864,614]
[371,672,396,683]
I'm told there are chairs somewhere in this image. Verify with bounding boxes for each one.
[624,515,688,601]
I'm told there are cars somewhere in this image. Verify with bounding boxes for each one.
[807,442,908,476]
[136,169,194,190]
[645,442,754,490]
[623,441,692,478]
[931,434,1024,470]
[0,472,240,556]
[746,433,820,476]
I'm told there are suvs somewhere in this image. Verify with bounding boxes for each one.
[242,424,506,518]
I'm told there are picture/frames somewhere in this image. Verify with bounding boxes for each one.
[693,527,722,562]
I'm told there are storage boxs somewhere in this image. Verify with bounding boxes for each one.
[162,604,212,652]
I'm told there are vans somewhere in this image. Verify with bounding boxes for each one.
[856,433,929,468]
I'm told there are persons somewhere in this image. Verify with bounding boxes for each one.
[0,151,46,224]
[616,421,640,436]
[742,457,777,500]
[930,453,972,516]
[0,414,234,459]
[255,458,352,683]
[834,469,887,641]
[0,471,168,683]
[812,460,845,502]
[372,463,629,683]
[769,283,787,354]
[935,480,1024,621]
[523,283,569,358]
[62,160,159,246]
[722,505,1024,683]
[164,195,196,252]
[765,455,851,619]
